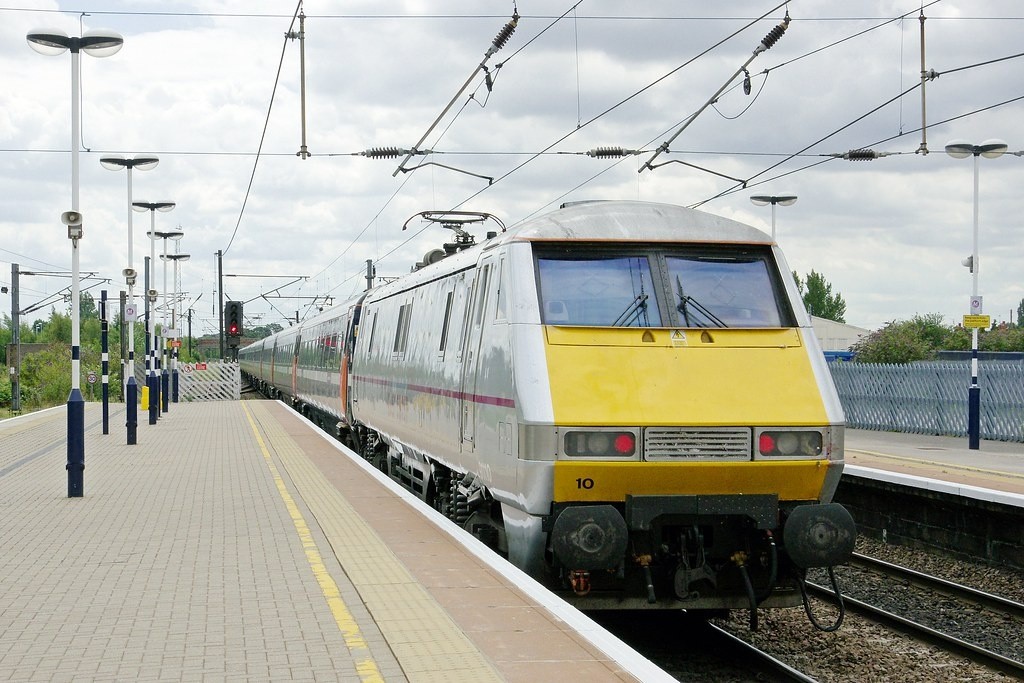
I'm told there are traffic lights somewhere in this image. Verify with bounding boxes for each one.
[225,300,244,335]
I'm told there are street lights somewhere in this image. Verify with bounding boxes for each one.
[145,227,191,413]
[132,200,176,425]
[748,194,798,239]
[945,137,1009,451]
[159,253,191,402]
[97,152,161,445]
[26,23,125,496]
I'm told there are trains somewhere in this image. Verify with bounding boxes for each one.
[233,198,858,638]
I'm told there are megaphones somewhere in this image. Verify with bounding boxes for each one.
[147,289,158,296]
[60,210,83,226]
[122,268,136,277]
